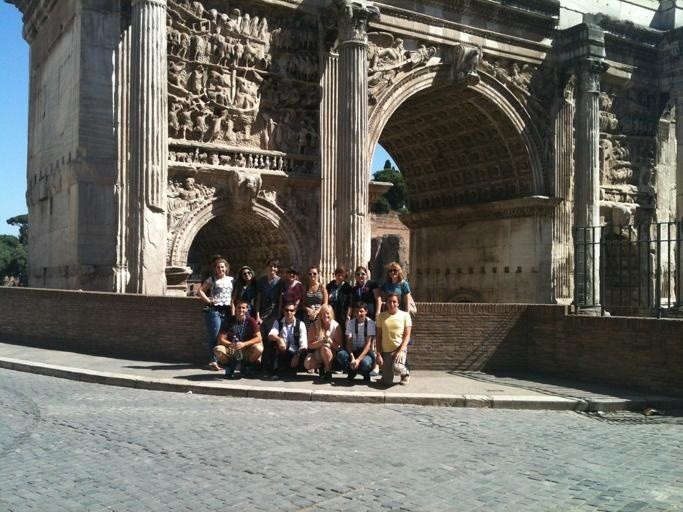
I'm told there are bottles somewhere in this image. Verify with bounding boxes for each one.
[232,336,243,361]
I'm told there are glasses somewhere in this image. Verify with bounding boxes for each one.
[285,308,294,312]
[355,272,365,276]
[244,272,250,275]
[309,272,316,275]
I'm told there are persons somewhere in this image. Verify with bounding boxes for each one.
[196,251,417,386]
